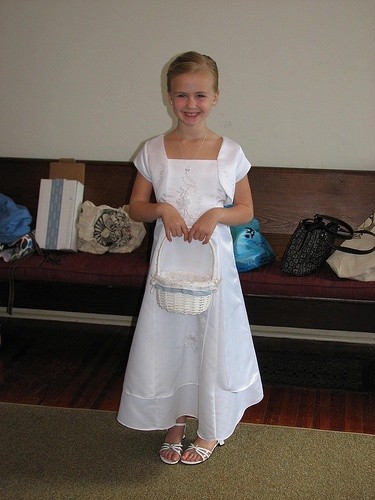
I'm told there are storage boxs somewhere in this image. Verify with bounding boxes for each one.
[34,178,84,253]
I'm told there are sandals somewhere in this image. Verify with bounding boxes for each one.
[159,422,186,465]
[181,439,224,465]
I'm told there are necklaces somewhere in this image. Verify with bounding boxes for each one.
[175,127,209,175]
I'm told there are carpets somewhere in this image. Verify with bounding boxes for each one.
[0,402,375,500]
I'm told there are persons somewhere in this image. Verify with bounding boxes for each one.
[116,50,265,466]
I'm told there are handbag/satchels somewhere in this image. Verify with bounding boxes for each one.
[77,200,147,255]
[280,214,375,277]
[224,205,276,273]
[0,193,34,261]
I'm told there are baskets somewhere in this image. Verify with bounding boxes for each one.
[150,229,221,316]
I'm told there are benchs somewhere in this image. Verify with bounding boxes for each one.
[0,157,375,300]
